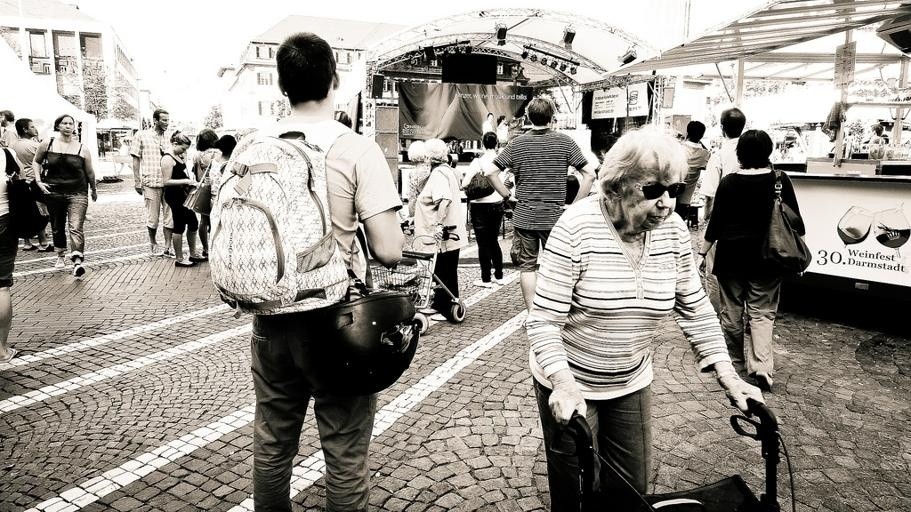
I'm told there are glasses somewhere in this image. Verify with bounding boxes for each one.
[634,182,688,199]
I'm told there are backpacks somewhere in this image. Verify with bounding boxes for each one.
[207,118,358,324]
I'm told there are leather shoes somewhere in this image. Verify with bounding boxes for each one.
[175,259,198,268]
[189,256,208,263]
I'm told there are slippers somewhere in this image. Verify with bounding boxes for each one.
[22,245,37,250]
[38,244,55,253]
[0,348,19,363]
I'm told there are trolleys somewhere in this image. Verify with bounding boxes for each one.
[546,396,796,512]
[371,236,467,337]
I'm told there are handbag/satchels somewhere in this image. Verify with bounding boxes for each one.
[465,173,494,201]
[766,170,812,278]
[183,162,212,217]
[30,138,46,204]
[3,147,50,239]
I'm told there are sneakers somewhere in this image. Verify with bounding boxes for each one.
[496,279,505,285]
[430,314,448,321]
[472,280,492,288]
[151,243,165,256]
[756,369,774,392]
[74,265,86,278]
[163,248,177,259]
[418,307,437,315]
[55,257,67,268]
[732,361,745,374]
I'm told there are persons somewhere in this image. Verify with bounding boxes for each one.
[10,118,50,253]
[485,90,598,314]
[228,33,411,512]
[160,129,208,269]
[406,141,432,219]
[775,123,891,165]
[0,110,18,154]
[695,129,808,391]
[193,127,218,256]
[31,114,101,280]
[1,147,29,363]
[462,131,508,288]
[482,112,496,135]
[672,120,711,224]
[208,134,238,208]
[701,107,747,321]
[129,109,175,258]
[496,114,512,143]
[560,175,580,210]
[446,153,462,188]
[413,138,470,322]
[524,126,765,509]
